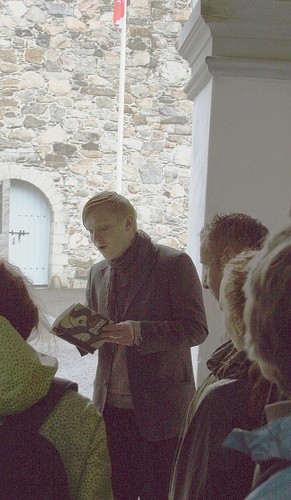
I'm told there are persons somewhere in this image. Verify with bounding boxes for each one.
[221,248,285,487]
[0,258,114,500]
[220,223,291,500]
[70,187,211,500]
[169,210,271,500]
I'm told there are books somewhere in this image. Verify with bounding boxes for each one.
[39,301,115,354]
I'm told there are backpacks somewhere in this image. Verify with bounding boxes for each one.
[1,378,80,500]
